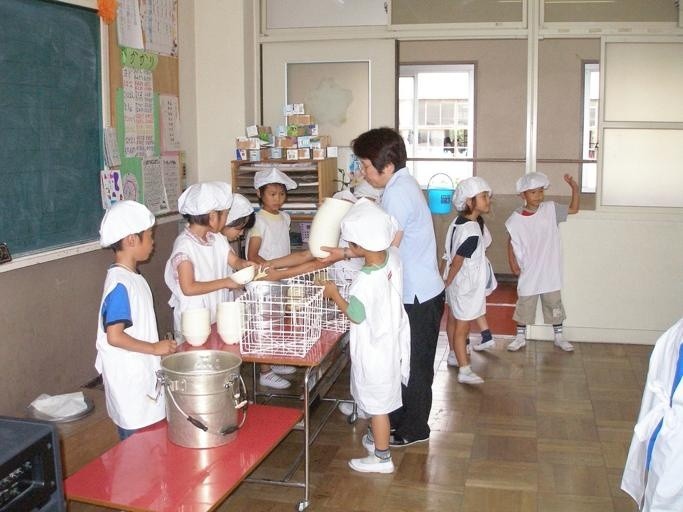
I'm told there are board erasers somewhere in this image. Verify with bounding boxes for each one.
[0,243,12,264]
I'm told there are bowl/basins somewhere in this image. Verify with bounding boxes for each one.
[308,196,354,259]
[227,265,256,286]
[215,302,246,346]
[179,308,212,347]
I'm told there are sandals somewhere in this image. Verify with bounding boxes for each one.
[390,431,429,447]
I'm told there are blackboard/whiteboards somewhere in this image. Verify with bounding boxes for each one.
[0,1,110,271]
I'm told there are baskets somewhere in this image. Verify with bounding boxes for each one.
[234,283,325,358]
[300,223,311,243]
[291,268,359,333]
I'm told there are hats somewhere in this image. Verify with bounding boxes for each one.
[225,193,254,226]
[100,201,155,248]
[333,191,357,203]
[517,172,550,196]
[254,168,297,190]
[354,183,378,199]
[178,182,232,216]
[340,198,398,252]
[452,177,492,212]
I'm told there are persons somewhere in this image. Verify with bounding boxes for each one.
[256,189,365,281]
[439,175,493,384]
[618,316,683,512]
[465,215,498,354]
[503,171,582,353]
[314,125,447,448]
[162,180,259,345]
[219,191,257,244]
[244,167,299,266]
[352,182,380,201]
[313,195,413,474]
[92,200,177,442]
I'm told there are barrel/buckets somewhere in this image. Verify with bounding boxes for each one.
[426,173,455,215]
[152,350,250,447]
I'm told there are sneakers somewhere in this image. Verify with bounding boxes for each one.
[339,403,371,419]
[362,434,375,453]
[473,340,495,352]
[447,353,458,366]
[259,372,291,389]
[554,334,575,351]
[507,336,527,352]
[466,345,473,355]
[348,456,394,473]
[458,372,484,384]
[270,365,296,374]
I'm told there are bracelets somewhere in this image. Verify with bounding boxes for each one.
[343,247,349,260]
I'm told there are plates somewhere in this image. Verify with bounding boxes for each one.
[26,397,96,423]
[246,319,286,330]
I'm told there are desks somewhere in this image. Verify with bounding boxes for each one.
[173,292,357,511]
[64,401,304,512]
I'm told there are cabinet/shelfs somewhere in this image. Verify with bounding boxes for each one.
[230,158,338,261]
